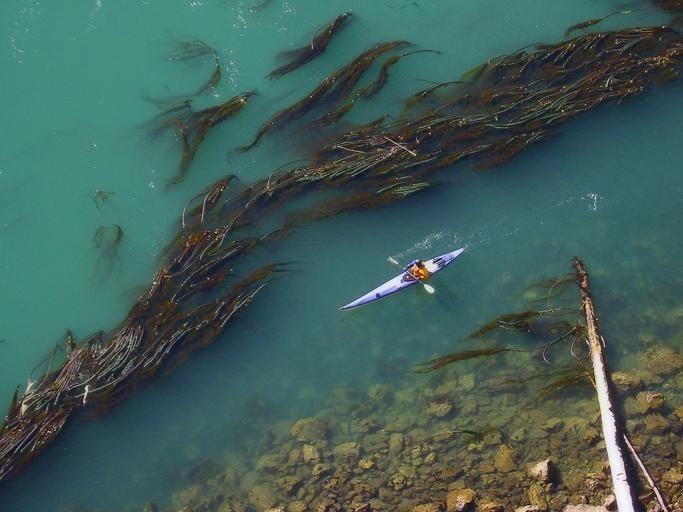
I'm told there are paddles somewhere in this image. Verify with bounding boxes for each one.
[386,255,435,294]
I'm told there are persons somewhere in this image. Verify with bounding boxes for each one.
[410,257,430,280]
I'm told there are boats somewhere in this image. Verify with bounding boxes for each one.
[338,244,471,312]
